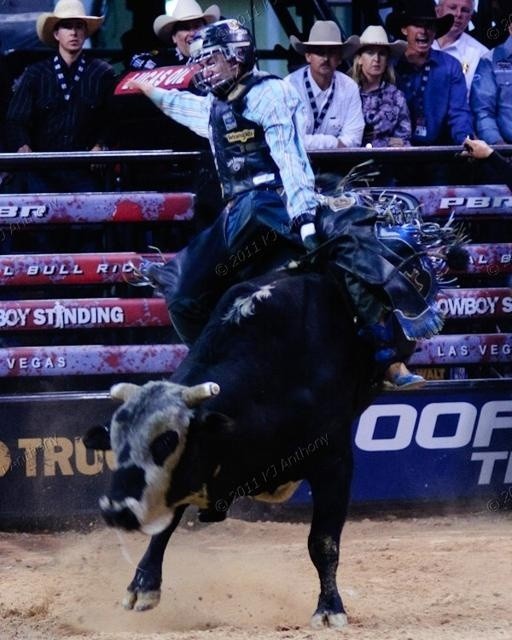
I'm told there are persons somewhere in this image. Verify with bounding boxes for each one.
[469,2,512,145]
[130,18,428,394]
[346,25,413,146]
[460,135,512,193]
[281,20,365,150]
[431,0,491,103]
[5,1,120,152]
[125,0,236,154]
[385,1,473,146]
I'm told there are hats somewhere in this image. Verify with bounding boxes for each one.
[289,18,361,54]
[385,1,455,38]
[153,1,222,36]
[360,25,408,51]
[35,1,106,43]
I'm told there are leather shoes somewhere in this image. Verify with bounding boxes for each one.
[385,372,426,393]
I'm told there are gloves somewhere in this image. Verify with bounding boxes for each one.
[300,231,333,267]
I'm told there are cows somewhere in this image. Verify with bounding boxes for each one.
[80,174,415,629]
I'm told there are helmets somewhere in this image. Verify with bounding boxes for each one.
[186,17,255,95]
